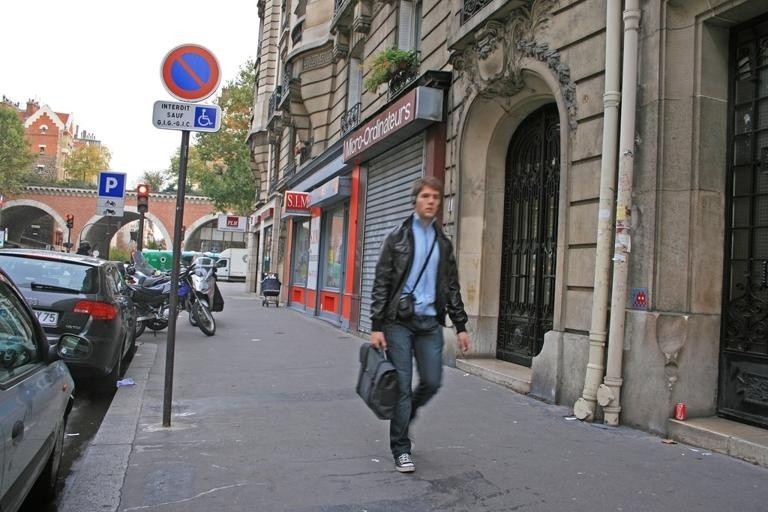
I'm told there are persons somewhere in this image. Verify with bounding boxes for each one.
[75,240,91,255]
[369,177,472,473]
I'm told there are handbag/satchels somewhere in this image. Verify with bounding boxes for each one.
[356,343,397,419]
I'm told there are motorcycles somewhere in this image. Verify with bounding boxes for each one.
[121,248,225,338]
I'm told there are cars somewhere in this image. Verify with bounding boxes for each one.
[0,264,93,512]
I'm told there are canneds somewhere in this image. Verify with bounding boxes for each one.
[674,402,687,421]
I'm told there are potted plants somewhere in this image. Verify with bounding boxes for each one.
[364,45,414,93]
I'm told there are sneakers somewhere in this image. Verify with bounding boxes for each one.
[395,453,416,472]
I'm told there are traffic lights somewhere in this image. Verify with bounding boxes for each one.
[181,225,185,240]
[66,214,74,228]
[130,231,138,242]
[137,184,149,212]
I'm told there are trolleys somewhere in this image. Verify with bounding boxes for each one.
[261,272,282,308]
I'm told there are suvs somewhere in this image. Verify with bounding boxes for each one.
[0,246,141,392]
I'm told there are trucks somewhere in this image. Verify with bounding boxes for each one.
[215,248,249,281]
